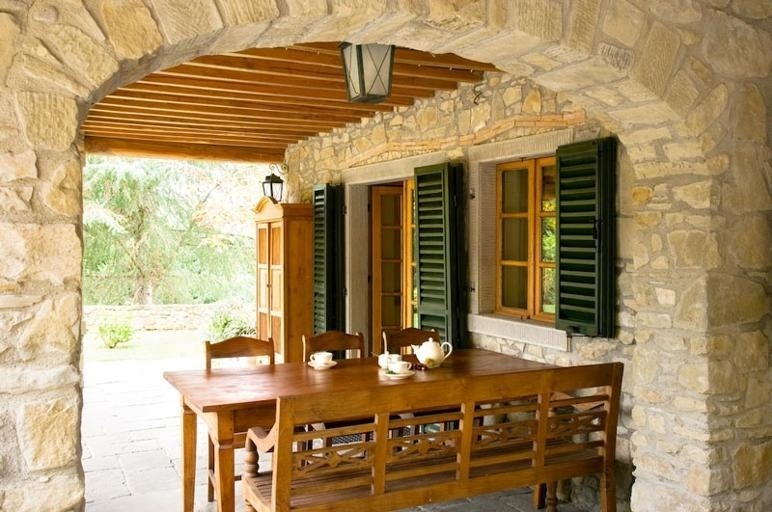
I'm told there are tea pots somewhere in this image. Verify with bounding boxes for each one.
[409,338,455,370]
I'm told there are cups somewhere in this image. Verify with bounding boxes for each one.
[391,361,413,374]
[310,349,333,365]
[379,351,402,369]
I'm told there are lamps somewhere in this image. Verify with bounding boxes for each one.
[260,161,290,204]
[337,41,395,106]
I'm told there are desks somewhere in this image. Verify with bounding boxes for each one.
[163,348,561,512]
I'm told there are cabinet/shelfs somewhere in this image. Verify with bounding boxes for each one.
[249,197,315,365]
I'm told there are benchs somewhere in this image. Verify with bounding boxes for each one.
[242,358,629,511]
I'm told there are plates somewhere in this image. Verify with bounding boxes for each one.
[382,372,417,379]
[307,361,337,371]
